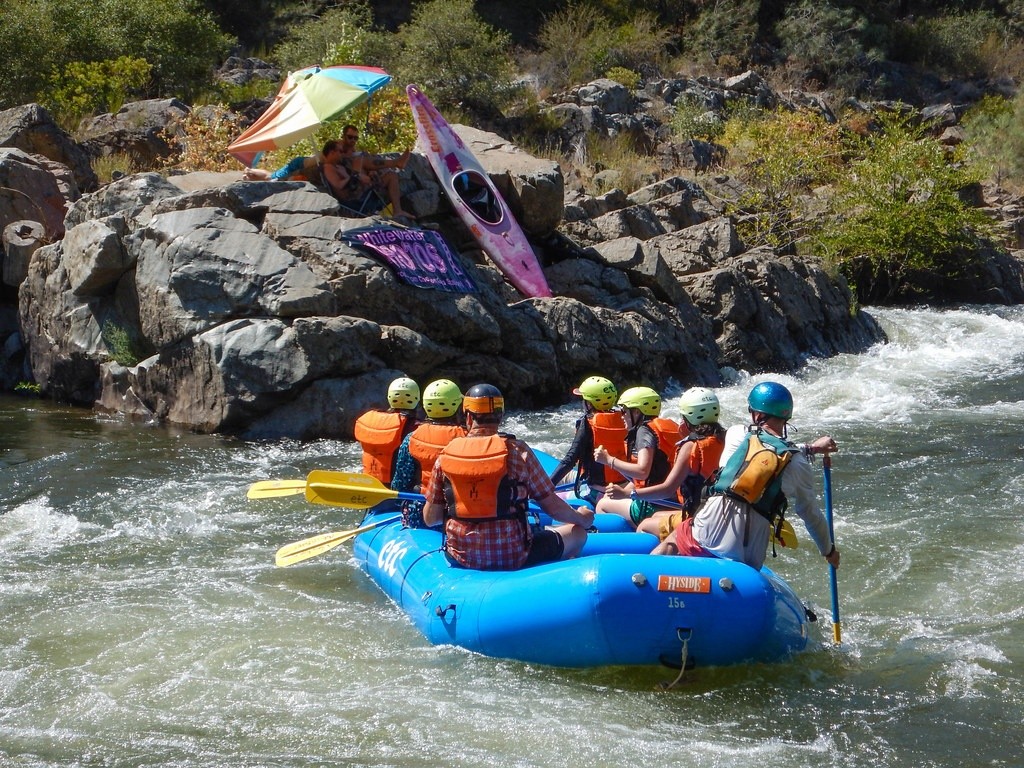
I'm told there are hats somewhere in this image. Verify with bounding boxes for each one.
[573,388,582,396]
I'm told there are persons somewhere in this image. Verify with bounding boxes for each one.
[607,387,725,541]
[549,376,627,499]
[594,387,680,527]
[320,140,416,219]
[423,383,593,571]
[355,377,421,512]
[649,382,840,572]
[243,124,359,182]
[391,379,469,529]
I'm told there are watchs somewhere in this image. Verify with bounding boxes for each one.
[630,489,638,501]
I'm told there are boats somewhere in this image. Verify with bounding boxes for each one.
[351,447,809,673]
[406,83,553,297]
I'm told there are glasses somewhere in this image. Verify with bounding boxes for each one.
[334,148,344,153]
[345,134,359,142]
[748,406,754,413]
[621,408,630,415]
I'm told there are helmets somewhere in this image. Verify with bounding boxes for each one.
[423,380,465,418]
[462,383,504,414]
[387,378,420,409]
[579,376,618,410]
[617,386,661,416]
[749,382,794,419]
[679,387,720,425]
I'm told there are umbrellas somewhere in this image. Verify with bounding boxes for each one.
[227,66,393,169]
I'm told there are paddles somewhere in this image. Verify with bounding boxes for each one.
[644,497,799,551]
[615,470,635,485]
[274,480,583,569]
[822,444,845,648]
[304,469,427,511]
[247,479,307,500]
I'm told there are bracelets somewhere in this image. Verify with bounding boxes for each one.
[611,457,616,469]
[805,444,814,461]
[827,544,835,558]
